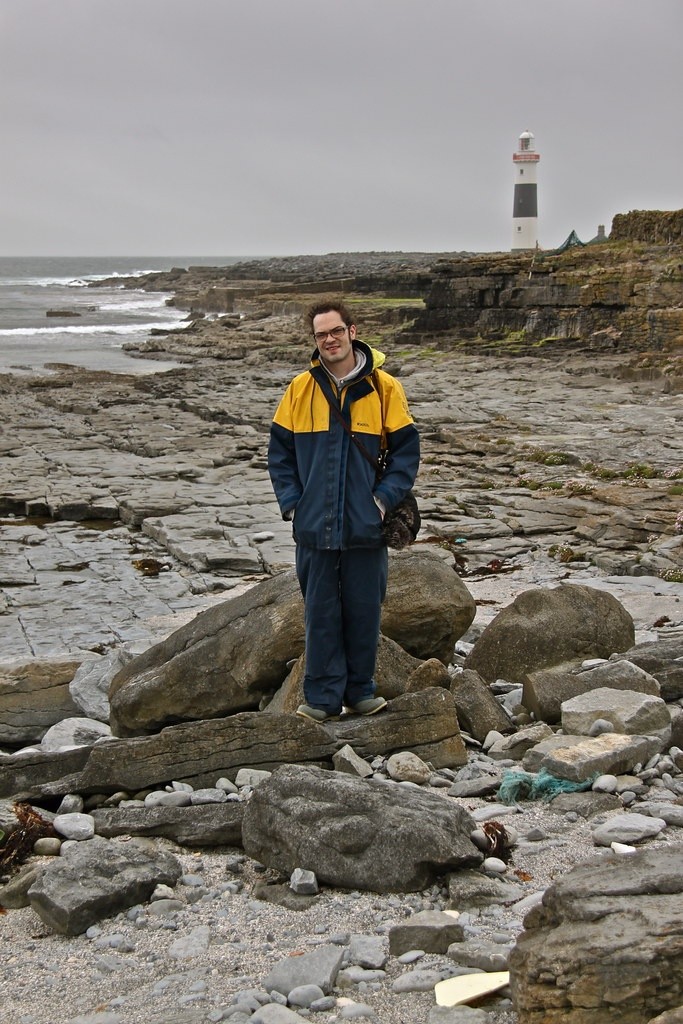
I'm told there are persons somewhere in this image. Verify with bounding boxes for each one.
[267,301,420,723]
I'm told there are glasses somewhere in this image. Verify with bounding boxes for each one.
[314,326,349,342]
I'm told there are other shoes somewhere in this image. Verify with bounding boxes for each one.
[296,705,340,724]
[344,697,388,716]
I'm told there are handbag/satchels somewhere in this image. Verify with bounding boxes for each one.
[374,447,421,550]
